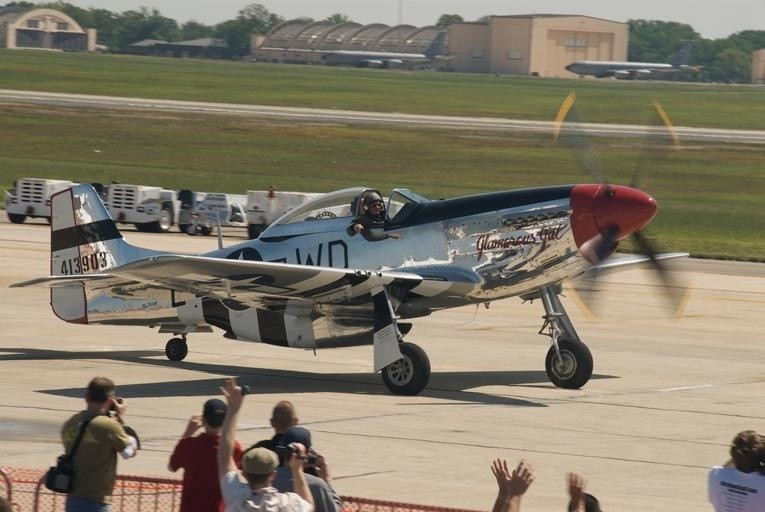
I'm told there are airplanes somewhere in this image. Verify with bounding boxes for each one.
[321,33,455,70]
[6,90,695,397]
[563,38,709,81]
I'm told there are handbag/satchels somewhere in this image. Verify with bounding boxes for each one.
[43,464,74,493]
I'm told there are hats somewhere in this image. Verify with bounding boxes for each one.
[240,446,279,477]
[281,426,311,449]
[203,399,226,418]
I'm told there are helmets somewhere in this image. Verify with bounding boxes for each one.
[365,192,382,205]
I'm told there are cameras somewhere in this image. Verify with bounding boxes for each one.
[110,397,123,411]
[274,446,293,467]
[307,456,320,466]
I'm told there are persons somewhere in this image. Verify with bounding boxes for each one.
[167,398,247,511]
[346,190,388,237]
[707,430,765,511]
[216,375,316,511]
[568,493,600,511]
[238,399,322,477]
[489,457,535,511]
[266,425,342,511]
[568,471,588,511]
[58,375,140,511]
